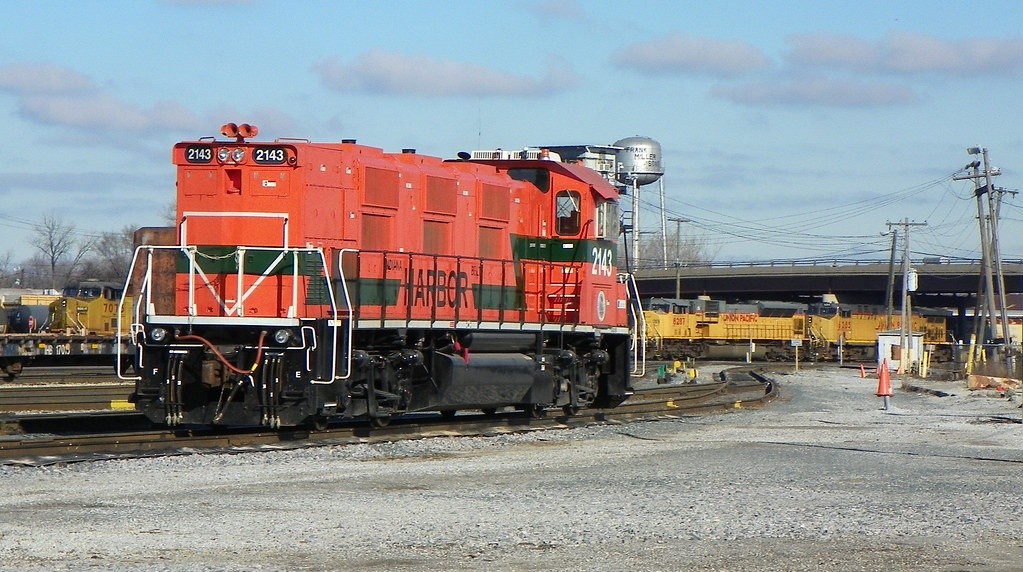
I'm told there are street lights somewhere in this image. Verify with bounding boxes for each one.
[880,230,896,329]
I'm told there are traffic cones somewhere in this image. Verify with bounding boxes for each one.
[883,357,892,389]
[859,363,868,379]
[875,366,881,379]
[874,363,895,397]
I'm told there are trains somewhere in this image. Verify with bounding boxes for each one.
[0,272,954,382]
[112,119,647,434]
